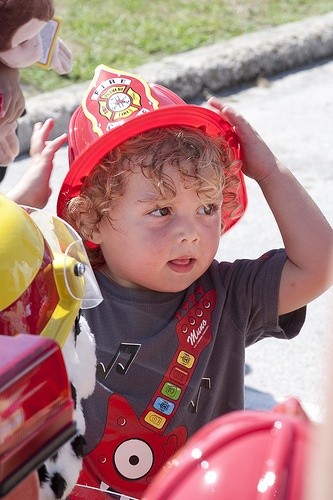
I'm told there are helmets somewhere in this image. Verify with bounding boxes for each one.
[138,397,322,500]
[55,64,249,251]
[0,195,85,351]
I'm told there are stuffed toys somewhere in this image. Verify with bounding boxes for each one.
[0,0,101,497]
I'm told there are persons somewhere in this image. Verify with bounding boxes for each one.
[5,80,333,500]
